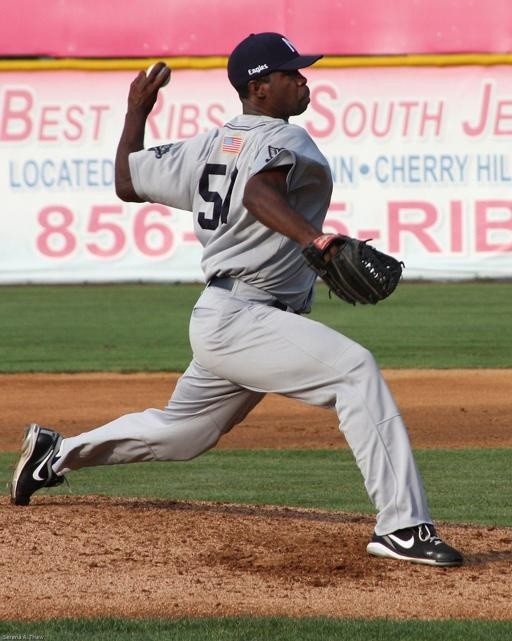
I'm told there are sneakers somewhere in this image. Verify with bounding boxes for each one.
[10,424,70,506]
[367,524,462,567]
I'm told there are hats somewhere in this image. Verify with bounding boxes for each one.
[228,32,323,90]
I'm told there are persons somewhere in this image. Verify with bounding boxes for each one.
[10,30,464,568]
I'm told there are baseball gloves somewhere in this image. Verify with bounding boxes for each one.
[301,233,406,306]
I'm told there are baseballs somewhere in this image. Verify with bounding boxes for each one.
[146,64,170,87]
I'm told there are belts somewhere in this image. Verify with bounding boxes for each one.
[208,276,236,290]
